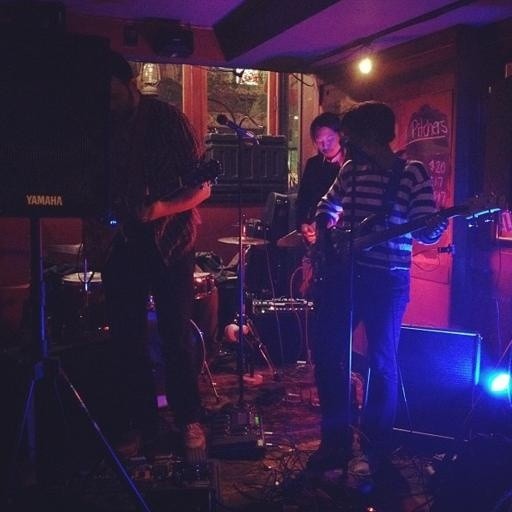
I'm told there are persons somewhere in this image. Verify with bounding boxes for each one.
[67,46,216,459]
[290,109,350,262]
[302,92,451,473]
[217,225,314,368]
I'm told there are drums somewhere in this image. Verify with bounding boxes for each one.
[141,311,206,394]
[59,271,105,335]
[193,272,219,299]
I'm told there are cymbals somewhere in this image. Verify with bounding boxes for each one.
[217,237,270,245]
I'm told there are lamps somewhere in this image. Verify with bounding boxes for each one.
[137,62,160,95]
[485,370,512,426]
[357,40,373,75]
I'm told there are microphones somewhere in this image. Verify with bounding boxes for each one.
[340,134,370,164]
[216,114,253,140]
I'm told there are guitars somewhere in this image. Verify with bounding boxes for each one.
[80,159,225,266]
[311,193,502,277]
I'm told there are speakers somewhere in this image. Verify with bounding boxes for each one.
[1,26,114,220]
[395,324,480,451]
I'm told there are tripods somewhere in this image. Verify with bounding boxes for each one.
[209,246,283,382]
[1,227,155,512]
